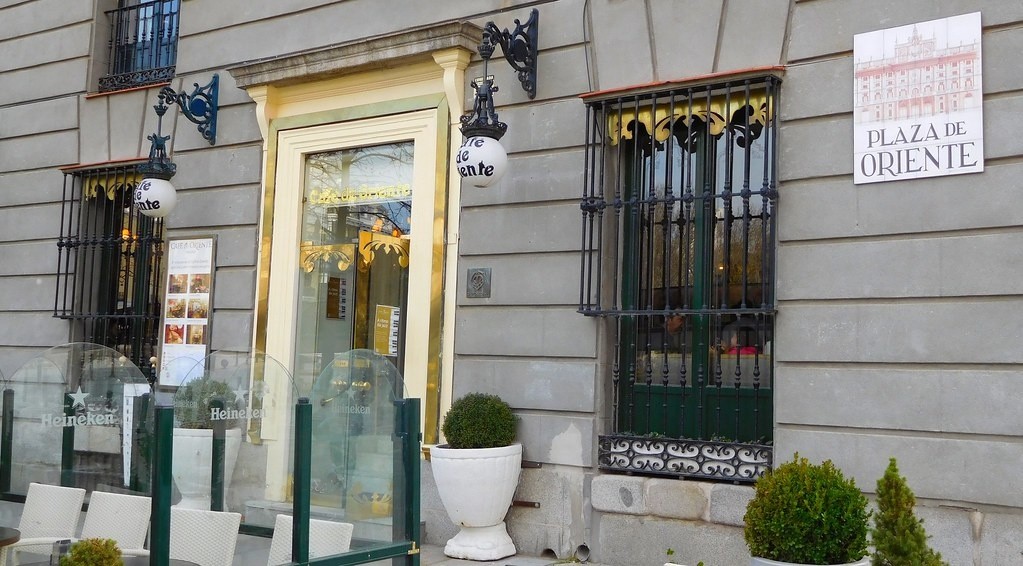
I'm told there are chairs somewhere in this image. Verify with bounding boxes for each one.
[82,491,152,559]
[267,514,353,566]
[170,506,242,566]
[0,483,86,562]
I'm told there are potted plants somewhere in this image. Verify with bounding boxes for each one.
[169,377,244,513]
[741,455,870,566]
[428,391,525,560]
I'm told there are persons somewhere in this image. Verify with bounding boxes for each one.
[647,303,689,351]
[716,299,769,354]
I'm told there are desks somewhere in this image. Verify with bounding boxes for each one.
[15,557,200,566]
[0,526,21,548]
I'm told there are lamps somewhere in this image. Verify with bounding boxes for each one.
[131,74,219,215]
[456,8,539,188]
